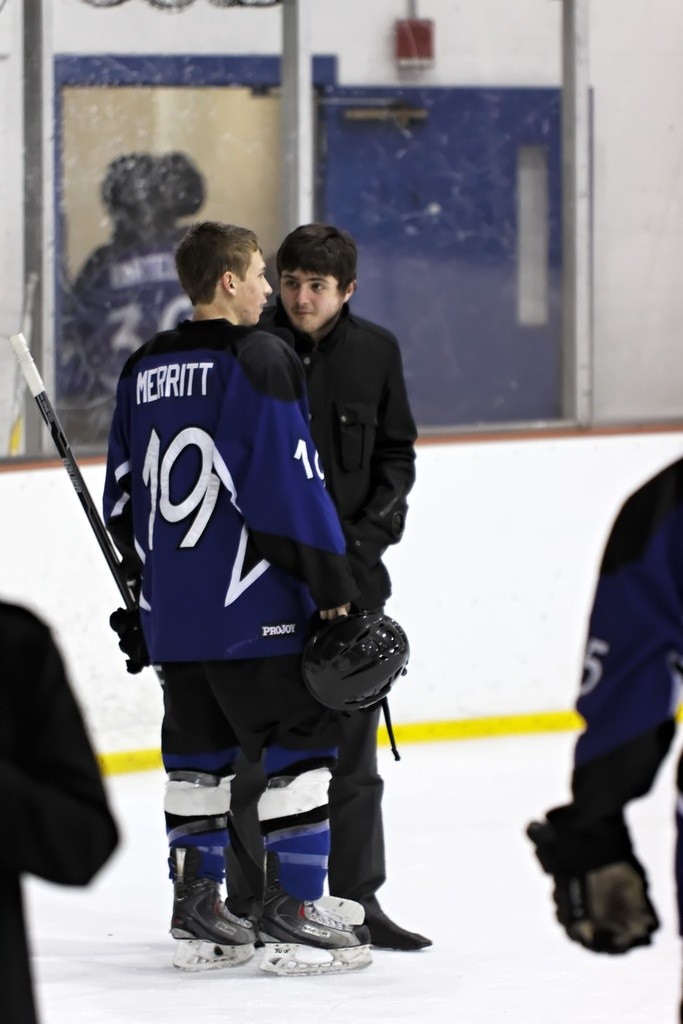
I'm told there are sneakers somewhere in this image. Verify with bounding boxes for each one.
[170,877,256,976]
[263,886,372,972]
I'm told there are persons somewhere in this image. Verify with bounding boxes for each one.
[227,222,434,952]
[102,219,374,973]
[525,454,683,1024]
[0,597,124,1024]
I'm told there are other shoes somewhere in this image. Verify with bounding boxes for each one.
[330,887,434,952]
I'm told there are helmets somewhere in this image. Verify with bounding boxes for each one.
[303,612,407,711]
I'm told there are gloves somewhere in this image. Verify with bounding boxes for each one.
[524,800,657,952]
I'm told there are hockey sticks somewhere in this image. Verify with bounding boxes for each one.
[8,332,167,692]
